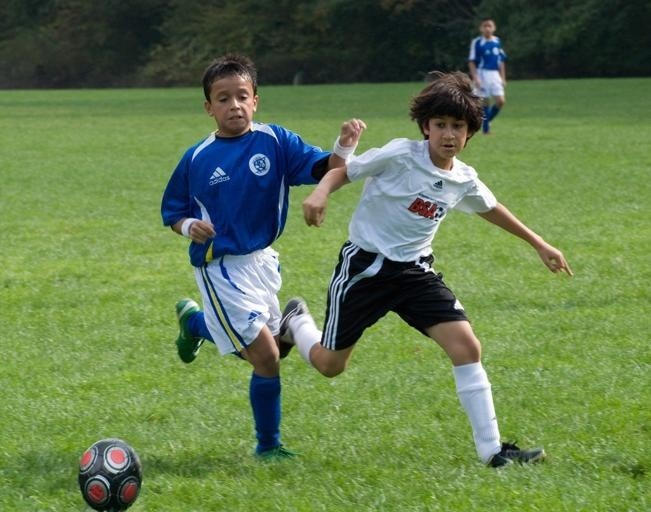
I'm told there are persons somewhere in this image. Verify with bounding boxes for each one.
[466,15,511,136]
[157,52,373,465]
[279,69,575,472]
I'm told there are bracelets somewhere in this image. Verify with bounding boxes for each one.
[182,218,198,239]
[330,136,361,161]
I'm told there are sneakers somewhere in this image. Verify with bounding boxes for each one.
[175,297,204,364]
[278,296,307,359]
[486,440,547,468]
[482,115,491,134]
[253,445,306,464]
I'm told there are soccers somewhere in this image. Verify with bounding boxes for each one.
[78,438,142,511]
